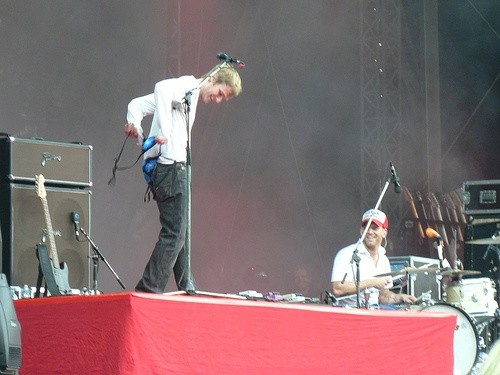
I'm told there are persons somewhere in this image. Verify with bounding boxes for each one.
[125,64,242,294]
[331,209,417,310]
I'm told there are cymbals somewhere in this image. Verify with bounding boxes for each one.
[432,271,482,276]
[374,268,444,278]
[464,234,500,245]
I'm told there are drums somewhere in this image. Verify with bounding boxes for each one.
[411,302,480,375]
[447,278,498,320]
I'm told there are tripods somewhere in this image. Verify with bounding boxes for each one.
[161,59,256,301]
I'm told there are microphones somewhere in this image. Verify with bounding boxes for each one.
[216,52,245,65]
[389,161,402,193]
[325,291,337,303]
[72,211,81,241]
[482,232,498,260]
[426,228,443,239]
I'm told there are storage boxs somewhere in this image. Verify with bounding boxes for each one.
[0,133,91,288]
[389,256,441,301]
[462,179,500,214]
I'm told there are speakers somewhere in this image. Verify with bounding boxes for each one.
[0,133,91,296]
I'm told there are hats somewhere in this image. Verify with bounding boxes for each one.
[361,208,388,229]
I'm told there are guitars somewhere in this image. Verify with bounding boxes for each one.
[34,174,70,296]
